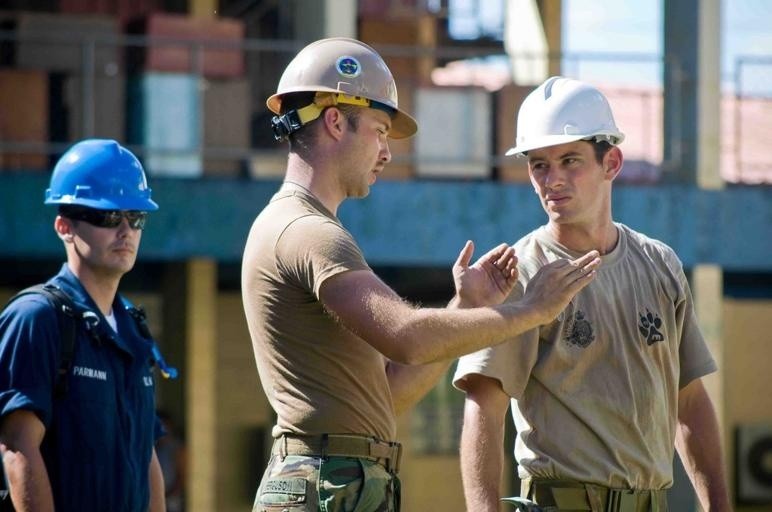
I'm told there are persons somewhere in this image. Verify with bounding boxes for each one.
[1,137,171,512]
[240,36,603,511]
[448,74,731,511]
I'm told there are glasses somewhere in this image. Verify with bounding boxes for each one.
[86,209,148,229]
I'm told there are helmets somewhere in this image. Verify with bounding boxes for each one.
[44,138,159,212]
[266,37,418,140]
[505,76,625,157]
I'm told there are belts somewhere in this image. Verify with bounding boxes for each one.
[274,433,402,476]
